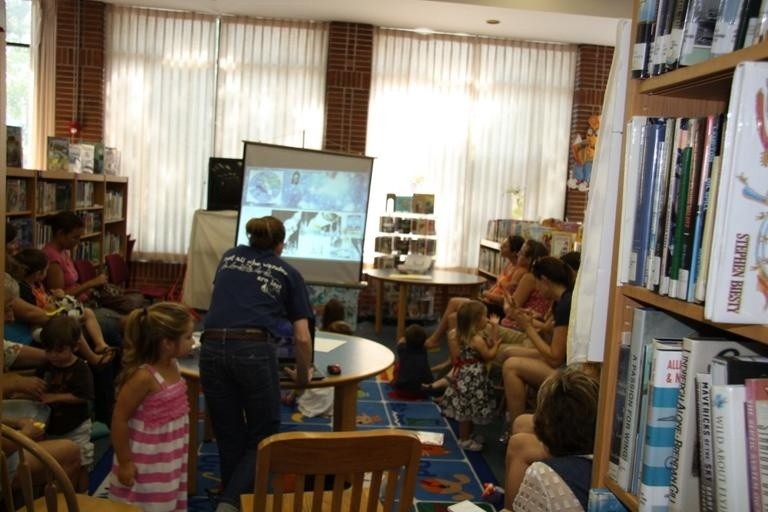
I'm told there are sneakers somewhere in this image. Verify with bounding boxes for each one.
[470,433,485,443]
[458,439,483,451]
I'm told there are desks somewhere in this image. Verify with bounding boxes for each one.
[161,325,398,496]
[364,263,488,340]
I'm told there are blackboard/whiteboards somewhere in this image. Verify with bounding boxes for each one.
[206,157,242,213]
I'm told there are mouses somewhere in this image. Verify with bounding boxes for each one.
[327,364,340,375]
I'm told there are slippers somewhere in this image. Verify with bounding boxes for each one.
[94,351,116,368]
[95,345,121,354]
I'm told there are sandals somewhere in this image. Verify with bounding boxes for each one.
[420,375,454,395]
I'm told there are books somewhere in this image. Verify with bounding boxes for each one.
[6,174,128,265]
[616,0,767,58]
[610,326,768,512]
[477,217,575,279]
[614,58,768,326]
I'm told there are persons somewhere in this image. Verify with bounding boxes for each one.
[295,320,351,421]
[319,298,345,331]
[198,217,313,511]
[388,234,602,511]
[1,212,195,511]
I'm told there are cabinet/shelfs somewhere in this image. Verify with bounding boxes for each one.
[6,166,129,269]
[475,220,581,293]
[588,1,768,512]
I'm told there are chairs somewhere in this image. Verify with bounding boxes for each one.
[1,421,142,511]
[73,259,98,284]
[105,254,165,305]
[239,427,423,511]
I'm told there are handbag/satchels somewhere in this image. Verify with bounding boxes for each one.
[44,294,85,320]
[100,284,144,313]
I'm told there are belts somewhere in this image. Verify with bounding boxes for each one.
[200,330,266,341]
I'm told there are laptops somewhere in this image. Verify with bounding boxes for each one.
[273,318,324,380]
